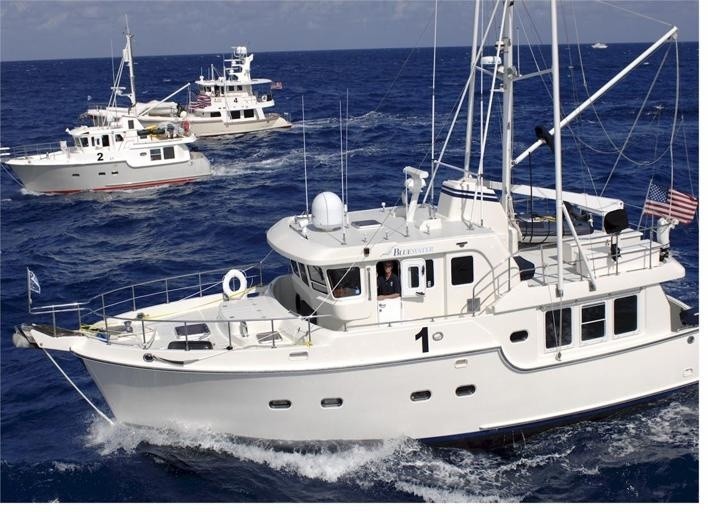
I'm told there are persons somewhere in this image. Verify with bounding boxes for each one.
[377,260,399,300]
[654,215,678,263]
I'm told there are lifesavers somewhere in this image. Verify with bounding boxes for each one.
[222,269,248,300]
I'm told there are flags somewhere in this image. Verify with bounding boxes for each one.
[28,270,41,294]
[645,183,696,224]
[270,80,283,89]
[188,82,211,110]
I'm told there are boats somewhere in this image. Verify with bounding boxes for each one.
[591,42,608,48]
[0,12,218,198]
[86,41,297,141]
[18,0,700,460]
